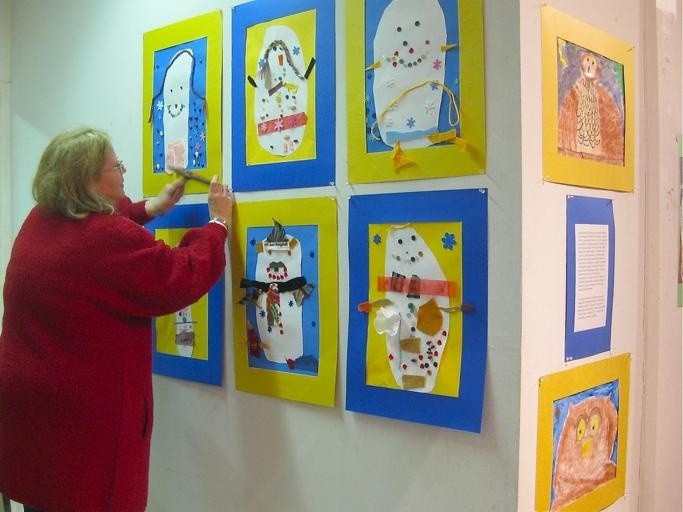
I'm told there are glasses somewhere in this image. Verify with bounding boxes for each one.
[89,159,123,176]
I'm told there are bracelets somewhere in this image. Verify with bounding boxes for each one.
[208,216,229,231]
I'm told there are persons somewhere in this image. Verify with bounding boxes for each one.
[0,125,235,511]
